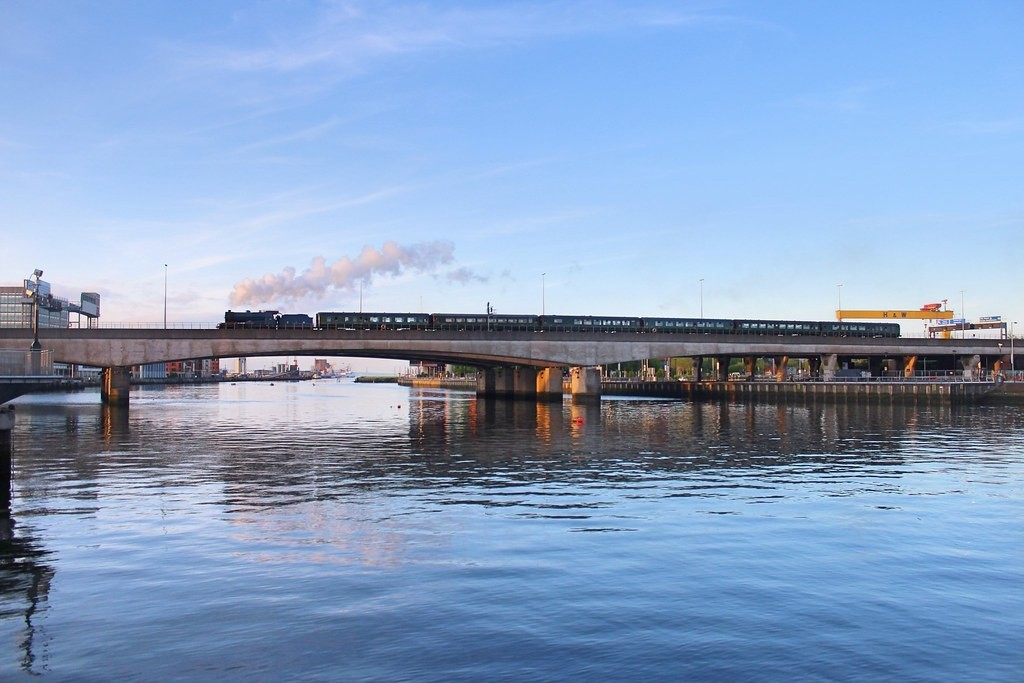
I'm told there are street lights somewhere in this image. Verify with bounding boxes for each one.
[164,264,167,329]
[700,279,704,319]
[1011,321,1017,382]
[542,273,546,315]
[960,291,965,339]
[836,284,843,310]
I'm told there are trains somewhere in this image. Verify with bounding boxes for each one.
[216,310,900,339]
